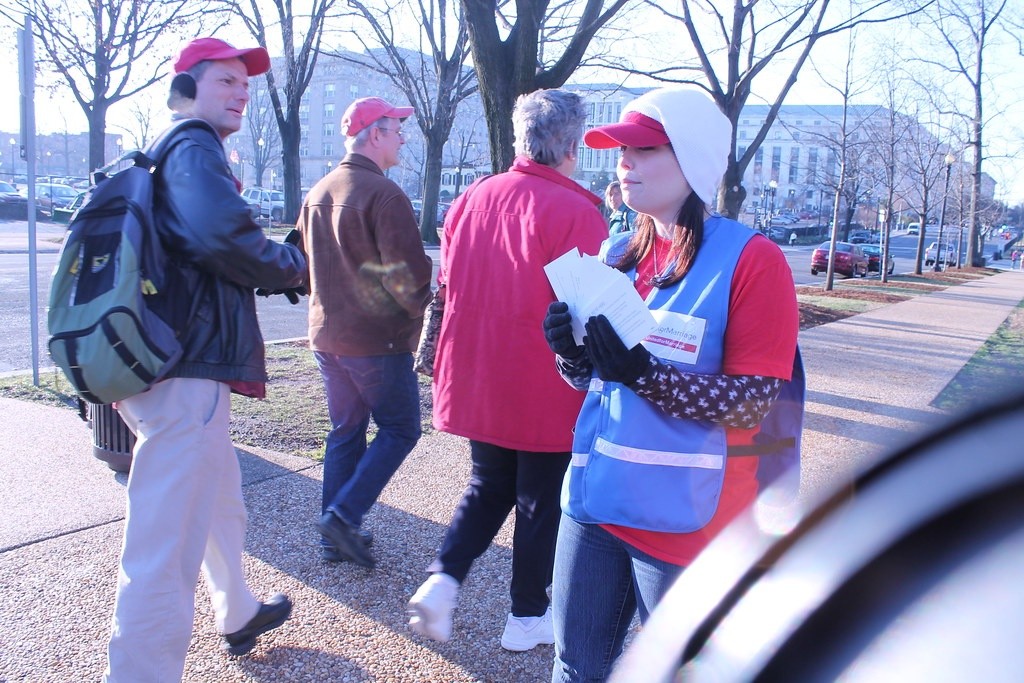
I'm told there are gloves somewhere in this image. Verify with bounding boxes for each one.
[581,316,643,385]
[542,302,585,359]
[256,229,306,304]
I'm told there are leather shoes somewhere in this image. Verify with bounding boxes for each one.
[224,595,293,654]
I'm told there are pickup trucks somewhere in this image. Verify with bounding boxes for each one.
[239,188,285,220]
[924,242,957,267]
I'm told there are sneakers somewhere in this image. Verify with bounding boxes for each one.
[407,576,456,642]
[320,529,373,560]
[316,512,376,567]
[501,606,555,651]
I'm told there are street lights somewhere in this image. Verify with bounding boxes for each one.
[9,138,16,183]
[117,138,123,171]
[454,167,459,198]
[791,191,796,213]
[257,138,265,187]
[766,180,778,238]
[328,162,332,173]
[932,149,957,271]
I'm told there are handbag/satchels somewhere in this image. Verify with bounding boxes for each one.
[412,285,443,376]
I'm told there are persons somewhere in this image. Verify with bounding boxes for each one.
[790,230,797,247]
[1011,250,1018,270]
[406,87,611,652]
[294,97,434,572]
[541,82,798,683]
[604,181,642,237]
[100,37,310,683]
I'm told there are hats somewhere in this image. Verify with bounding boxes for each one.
[341,97,415,135]
[174,38,270,77]
[583,111,670,147]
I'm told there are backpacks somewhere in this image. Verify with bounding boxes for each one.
[48,119,224,404]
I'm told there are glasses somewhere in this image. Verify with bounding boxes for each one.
[378,128,411,142]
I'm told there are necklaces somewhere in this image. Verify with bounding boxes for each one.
[653,234,657,275]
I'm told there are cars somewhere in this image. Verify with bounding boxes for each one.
[856,244,895,274]
[765,227,787,239]
[743,205,820,228]
[247,203,261,219]
[811,241,869,278]
[10,174,89,194]
[411,199,454,227]
[0,180,28,219]
[851,230,890,244]
[300,187,311,204]
[18,183,79,218]
[907,222,920,236]
